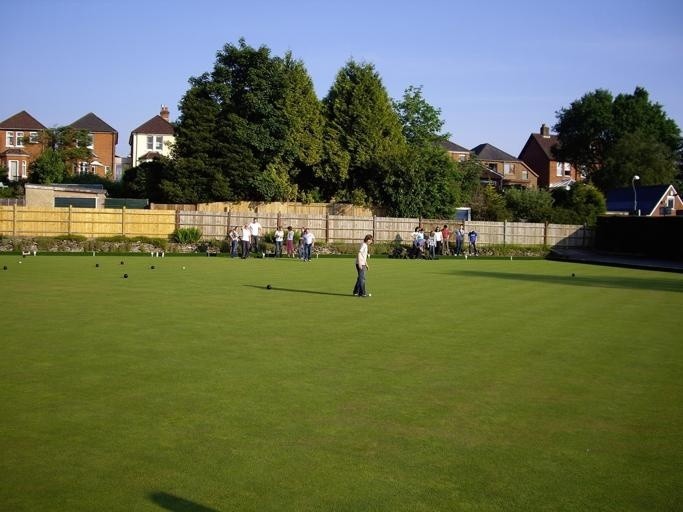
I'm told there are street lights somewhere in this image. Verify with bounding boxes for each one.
[630,174,640,209]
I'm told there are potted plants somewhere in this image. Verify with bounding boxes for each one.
[259,230,301,258]
[169,226,204,254]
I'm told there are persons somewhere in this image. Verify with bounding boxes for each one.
[228,217,316,262]
[410,224,479,260]
[353,234,374,297]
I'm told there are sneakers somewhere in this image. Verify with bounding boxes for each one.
[303,259,312,262]
[353,292,372,297]
[454,253,479,257]
